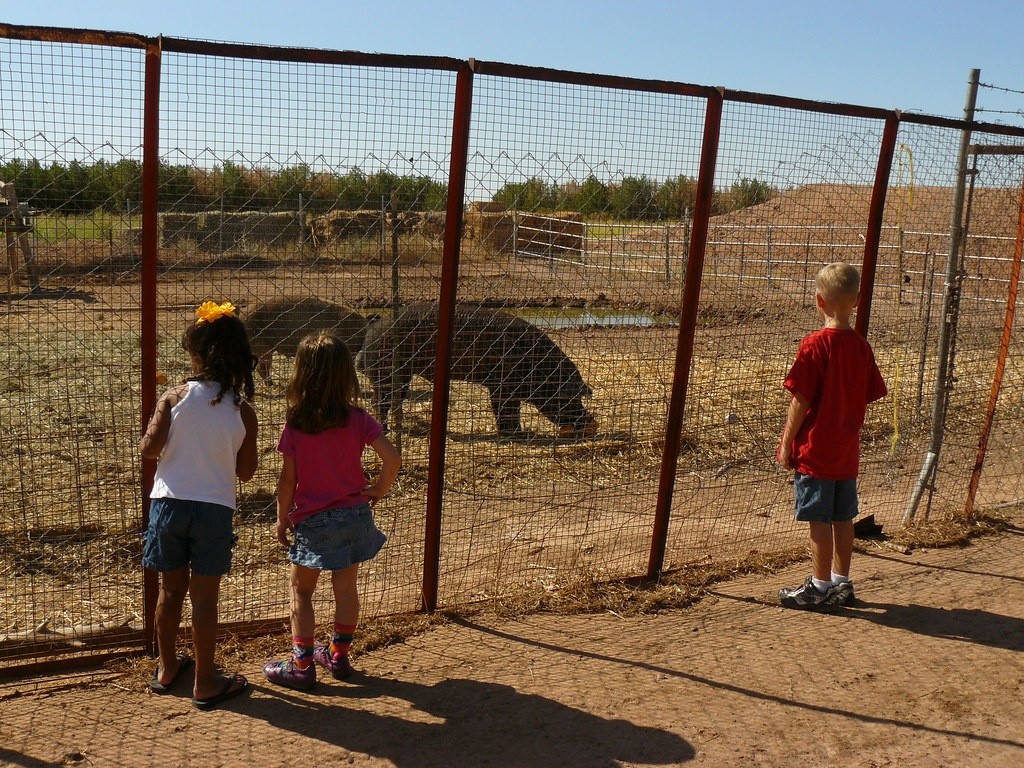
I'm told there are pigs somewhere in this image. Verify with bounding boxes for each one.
[240,293,381,385]
[356,304,596,436]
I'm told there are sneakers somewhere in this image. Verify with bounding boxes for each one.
[777,577,854,612]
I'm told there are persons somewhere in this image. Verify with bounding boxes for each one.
[140,300,259,709]
[777,260,888,614]
[263,329,402,691]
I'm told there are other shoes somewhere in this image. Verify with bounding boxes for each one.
[311,644,351,678]
[264,657,316,691]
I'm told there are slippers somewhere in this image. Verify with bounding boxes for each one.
[193,673,249,709]
[150,652,189,695]
